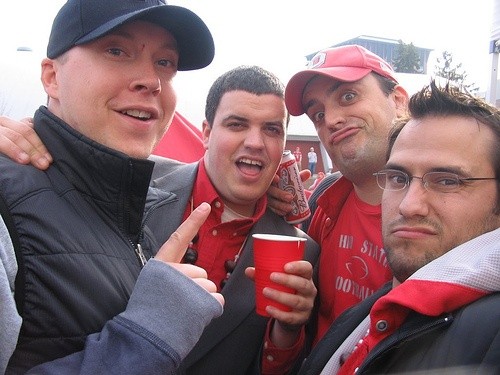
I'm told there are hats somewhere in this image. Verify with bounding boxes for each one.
[46,0,215,72]
[284,45,399,116]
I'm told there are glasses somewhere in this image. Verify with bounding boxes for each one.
[373,169,497,194]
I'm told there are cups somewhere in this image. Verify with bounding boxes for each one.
[252,234,309,316]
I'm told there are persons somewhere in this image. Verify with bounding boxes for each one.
[266,44,409,375]
[293,146,302,172]
[0,0,226,375]
[297,76,500,375]
[309,172,325,191]
[308,147,317,176]
[0,65,322,375]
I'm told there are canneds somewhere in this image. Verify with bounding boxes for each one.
[272,150,311,224]
